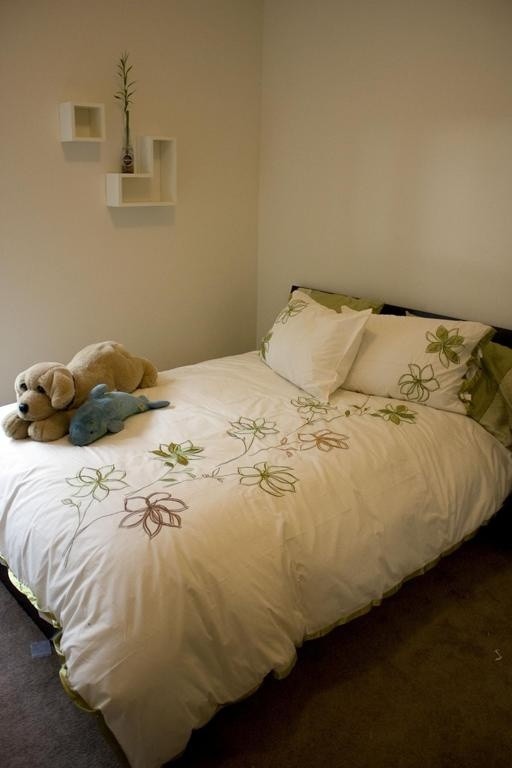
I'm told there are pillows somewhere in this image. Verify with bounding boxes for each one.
[256,289,498,415]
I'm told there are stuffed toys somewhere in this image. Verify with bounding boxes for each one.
[1,340,159,441]
[69,383,171,447]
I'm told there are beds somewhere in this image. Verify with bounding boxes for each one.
[0,283,511,666]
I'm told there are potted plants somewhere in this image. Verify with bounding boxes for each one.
[112,46,139,173]
